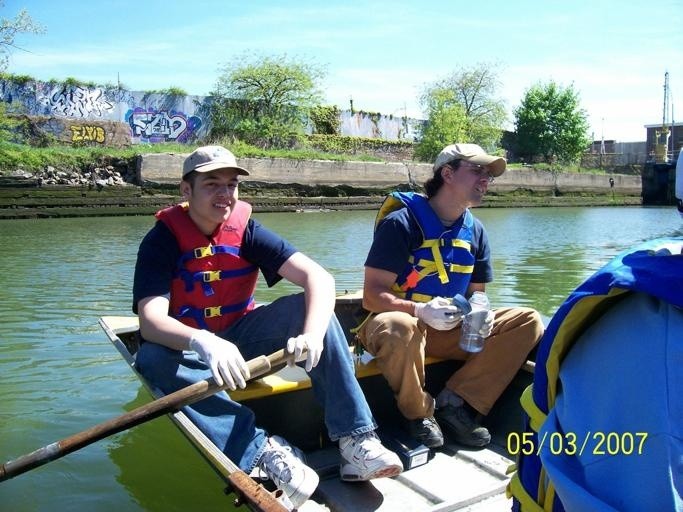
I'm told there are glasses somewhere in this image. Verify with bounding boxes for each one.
[450,162,496,183]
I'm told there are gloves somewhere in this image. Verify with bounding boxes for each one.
[285,333,325,373]
[459,308,496,339]
[414,296,465,332]
[189,329,251,392]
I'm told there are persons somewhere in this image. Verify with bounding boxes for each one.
[130,145,404,509]
[609,177,615,189]
[356,143,545,447]
[505,142,683,512]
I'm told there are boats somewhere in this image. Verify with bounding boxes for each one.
[95,287,558,511]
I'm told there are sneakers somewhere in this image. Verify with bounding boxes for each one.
[433,393,493,448]
[257,434,321,510]
[408,413,445,450]
[336,431,405,484]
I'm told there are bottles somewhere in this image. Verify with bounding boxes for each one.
[459,291,491,353]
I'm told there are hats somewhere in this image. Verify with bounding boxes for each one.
[181,144,251,182]
[432,142,507,178]
